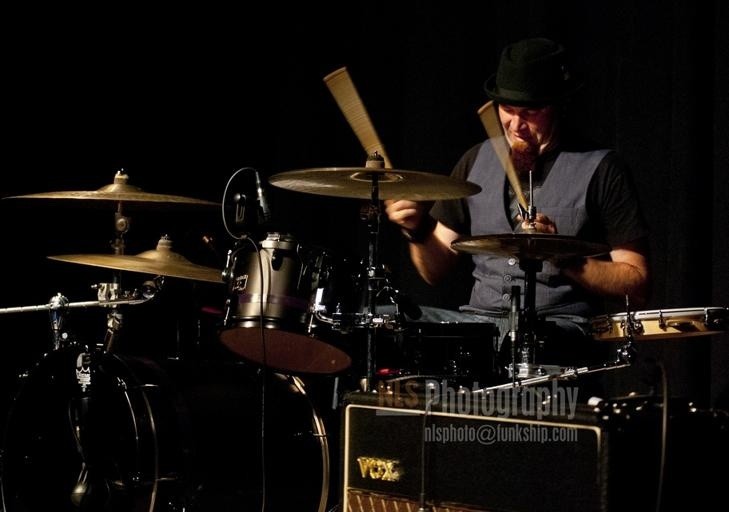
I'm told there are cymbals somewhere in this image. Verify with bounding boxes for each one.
[271,168,482,202]
[7,178,216,210]
[450,231,615,260]
[48,246,228,286]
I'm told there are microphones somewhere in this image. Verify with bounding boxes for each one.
[255,171,273,223]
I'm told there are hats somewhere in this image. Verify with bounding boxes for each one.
[484,38,561,107]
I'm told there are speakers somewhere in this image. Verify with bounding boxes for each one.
[338,391,617,512]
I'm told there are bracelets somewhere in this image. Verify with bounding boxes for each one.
[402,213,437,244]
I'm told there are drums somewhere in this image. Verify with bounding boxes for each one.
[220,228,381,379]
[587,304,729,347]
[473,358,644,455]
[3,344,330,512]
[332,314,500,418]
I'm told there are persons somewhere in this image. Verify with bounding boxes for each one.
[382,41,653,399]
